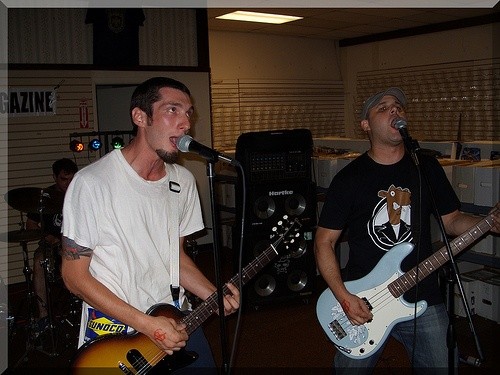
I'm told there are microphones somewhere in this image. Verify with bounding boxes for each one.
[175,135,234,163]
[393,118,419,152]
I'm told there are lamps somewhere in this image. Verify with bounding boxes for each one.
[70,133,85,153]
[110,130,124,149]
[88,131,102,151]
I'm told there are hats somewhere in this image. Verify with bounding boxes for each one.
[361,86,407,120]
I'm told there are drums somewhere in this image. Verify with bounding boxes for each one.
[43,242,71,303]
[183,237,199,263]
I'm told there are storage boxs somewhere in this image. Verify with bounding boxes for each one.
[219,137,500,324]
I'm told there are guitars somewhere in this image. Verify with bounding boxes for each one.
[68,218,306,375]
[316,205,500,360]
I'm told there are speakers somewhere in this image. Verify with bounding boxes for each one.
[238,172,318,301]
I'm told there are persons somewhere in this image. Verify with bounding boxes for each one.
[314,87,500,375]
[61,77,239,375]
[26,158,78,334]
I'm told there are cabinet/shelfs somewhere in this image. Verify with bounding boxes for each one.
[448,201,500,375]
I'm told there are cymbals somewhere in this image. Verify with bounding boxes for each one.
[0,229,48,243]
[4,187,65,215]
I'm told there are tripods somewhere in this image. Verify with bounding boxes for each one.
[6,197,84,359]
